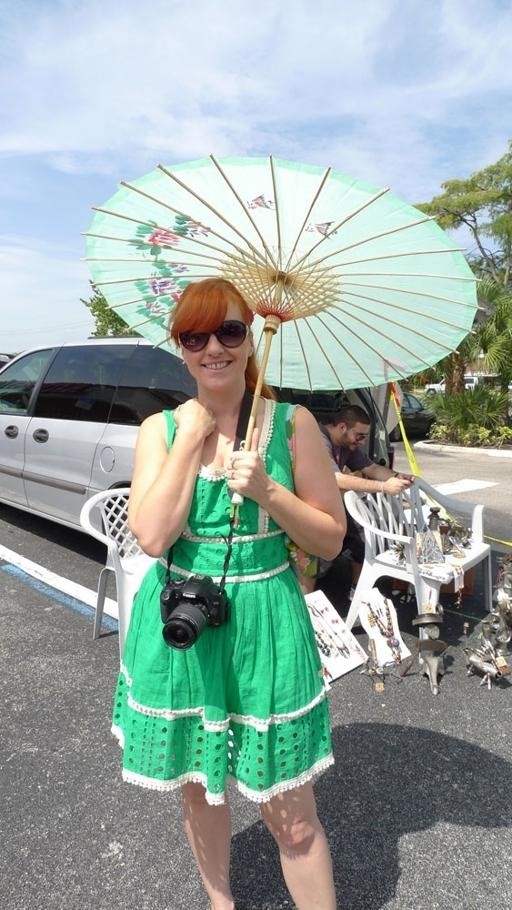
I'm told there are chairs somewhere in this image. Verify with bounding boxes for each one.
[343,473,493,667]
[79,487,161,665]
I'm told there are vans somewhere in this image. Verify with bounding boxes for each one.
[0,328,408,567]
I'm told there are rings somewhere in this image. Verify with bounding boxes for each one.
[231,470,236,480]
[231,459,239,470]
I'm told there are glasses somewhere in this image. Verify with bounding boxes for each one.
[356,433,368,443]
[179,321,250,352]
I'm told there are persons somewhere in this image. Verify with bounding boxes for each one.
[296,405,416,611]
[108,277,347,910]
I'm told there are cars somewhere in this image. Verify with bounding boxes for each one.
[0,353,10,370]
[386,389,437,444]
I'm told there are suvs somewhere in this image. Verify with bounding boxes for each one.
[423,373,480,397]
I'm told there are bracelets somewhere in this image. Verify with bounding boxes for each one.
[393,472,399,476]
[380,480,385,495]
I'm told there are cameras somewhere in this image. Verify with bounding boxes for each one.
[160,574,230,651]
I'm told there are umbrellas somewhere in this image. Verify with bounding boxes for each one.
[82,154,488,510]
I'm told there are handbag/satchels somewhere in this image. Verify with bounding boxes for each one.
[285,539,318,578]
